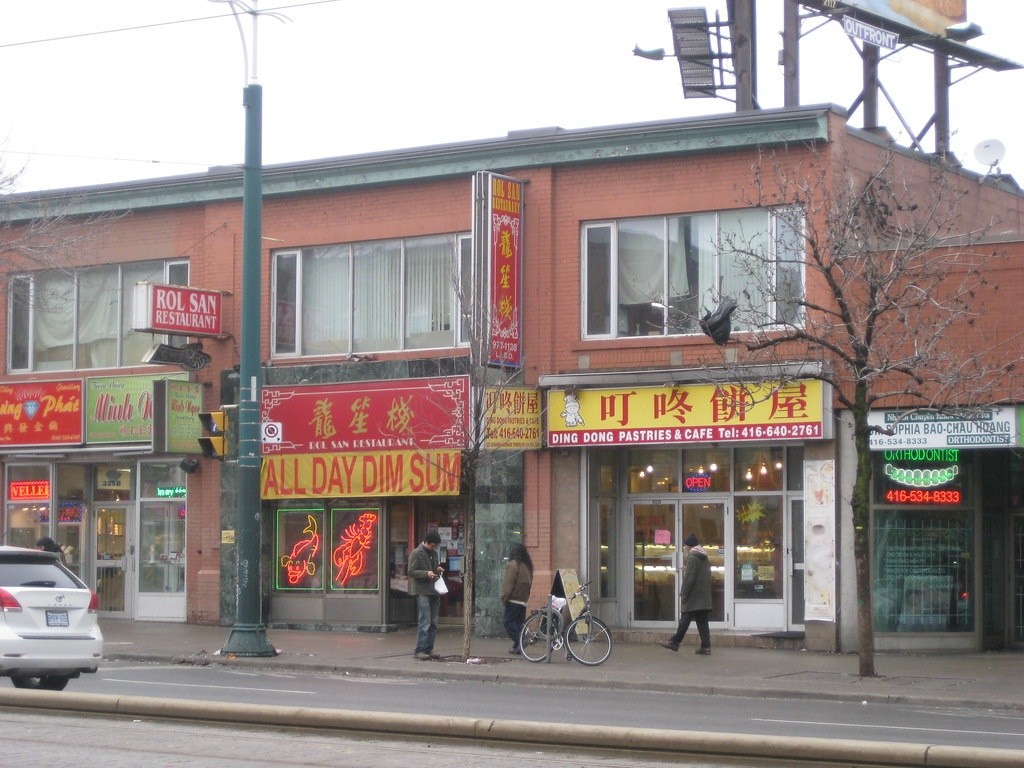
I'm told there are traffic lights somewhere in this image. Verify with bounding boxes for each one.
[196,409,226,462]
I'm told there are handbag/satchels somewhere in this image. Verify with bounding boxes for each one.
[434,571,449,595]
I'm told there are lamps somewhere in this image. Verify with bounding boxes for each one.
[943,22,983,42]
[179,458,196,474]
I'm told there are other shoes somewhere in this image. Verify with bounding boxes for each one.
[414,650,430,659]
[695,646,711,655]
[660,639,680,651]
[510,641,528,653]
[428,649,440,658]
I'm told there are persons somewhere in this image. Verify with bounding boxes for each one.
[659,531,713,655]
[37,537,67,567]
[406,530,445,661]
[499,542,534,654]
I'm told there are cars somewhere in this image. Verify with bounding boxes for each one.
[0,545,104,691]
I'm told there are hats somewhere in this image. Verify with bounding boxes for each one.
[685,533,698,547]
[425,529,441,543]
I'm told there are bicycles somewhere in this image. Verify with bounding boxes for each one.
[518,580,614,667]
